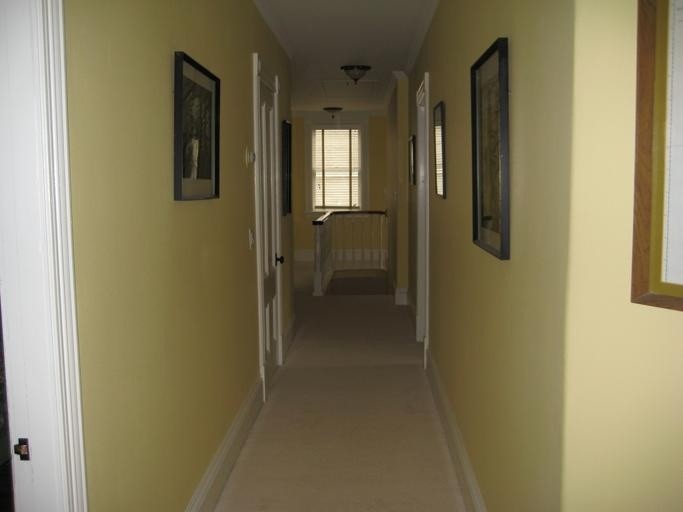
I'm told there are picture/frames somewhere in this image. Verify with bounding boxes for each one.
[174,51,220,201]
[470,36,511,261]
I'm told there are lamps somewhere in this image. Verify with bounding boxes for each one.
[340,65,371,85]
[324,107,342,120]
